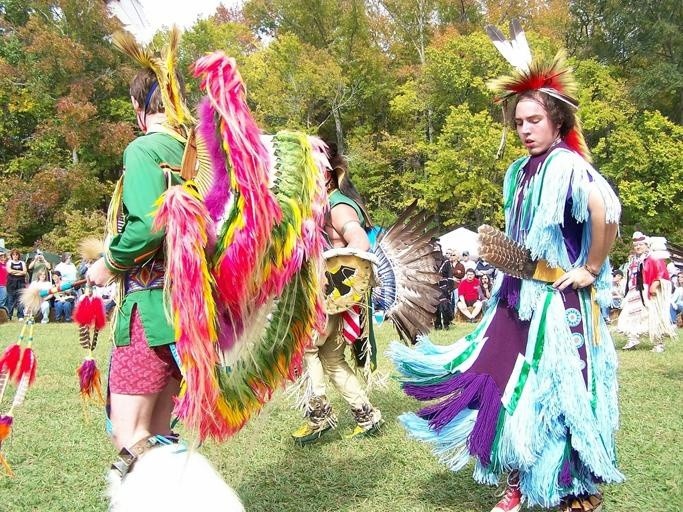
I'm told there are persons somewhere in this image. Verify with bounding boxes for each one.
[86,67,198,454]
[292,153,383,444]
[0,251,116,323]
[386,90,626,512]
[429,228,683,353]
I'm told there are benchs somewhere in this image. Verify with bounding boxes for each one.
[33,307,65,322]
[455,308,484,323]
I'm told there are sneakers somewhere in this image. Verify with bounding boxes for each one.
[291,395,338,443]
[621,336,641,350]
[344,403,387,441]
[489,471,526,512]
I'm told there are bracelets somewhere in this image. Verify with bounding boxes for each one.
[584,265,600,279]
